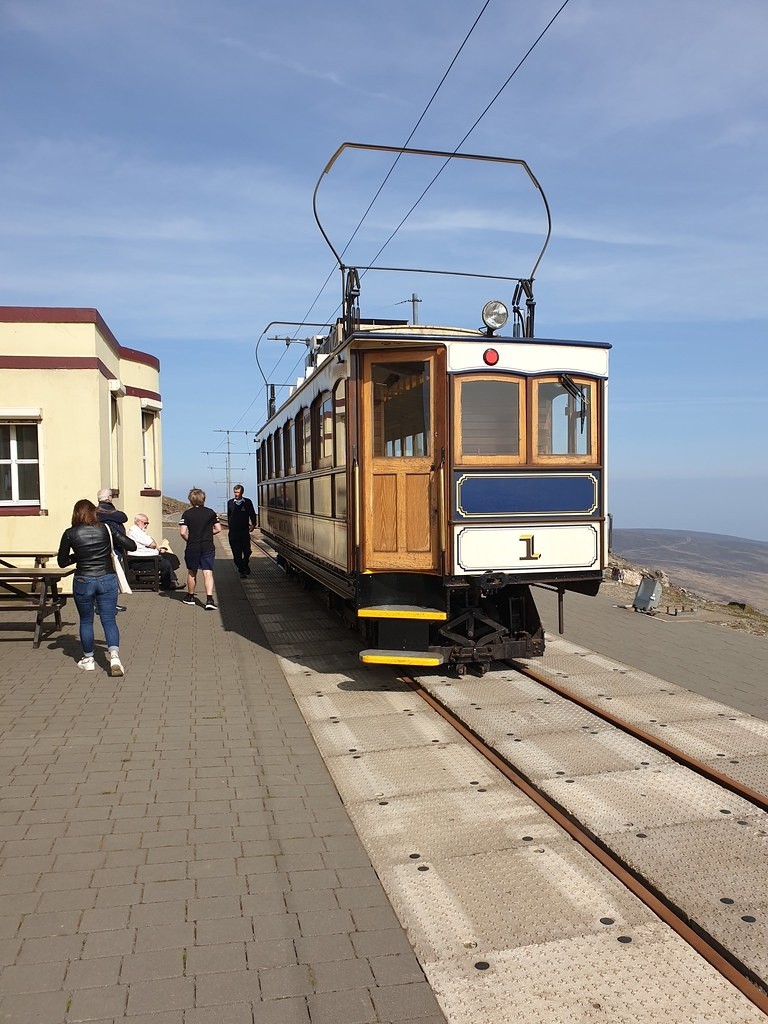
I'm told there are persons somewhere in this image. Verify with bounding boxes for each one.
[180,489,221,609]
[57,499,137,676]
[128,513,186,590]
[268,486,292,526]
[227,485,256,578]
[97,490,128,613]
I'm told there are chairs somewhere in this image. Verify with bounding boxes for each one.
[121,549,162,592]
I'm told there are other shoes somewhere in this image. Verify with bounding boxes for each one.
[240,570,246,578]
[116,606,126,611]
[95,608,118,615]
[169,580,186,589]
[243,559,250,575]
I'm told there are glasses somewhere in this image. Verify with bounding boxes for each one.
[138,520,149,525]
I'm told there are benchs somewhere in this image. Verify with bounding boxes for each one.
[0,577,45,584]
[0,593,74,606]
[0,604,61,613]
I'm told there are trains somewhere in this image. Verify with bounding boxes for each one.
[255,143,615,672]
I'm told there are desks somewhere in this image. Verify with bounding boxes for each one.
[0,550,58,605]
[0,568,76,650]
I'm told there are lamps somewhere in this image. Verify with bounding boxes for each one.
[633,578,662,610]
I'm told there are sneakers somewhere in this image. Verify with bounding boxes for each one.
[182,594,196,604]
[205,600,218,610]
[110,658,124,676]
[77,656,95,670]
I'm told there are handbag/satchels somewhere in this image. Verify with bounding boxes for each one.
[104,524,132,595]
[159,547,180,570]
